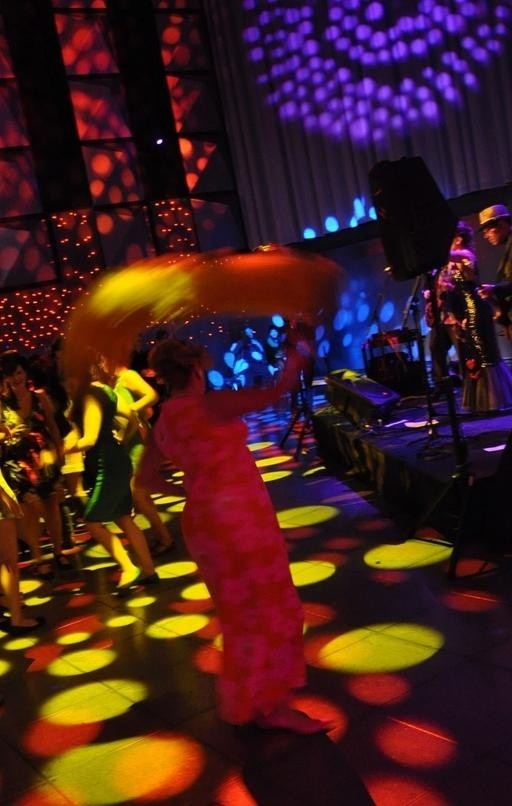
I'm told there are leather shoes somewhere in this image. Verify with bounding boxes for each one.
[110,571,162,594]
[150,538,178,559]
[53,551,70,567]
[5,615,48,632]
[36,559,55,581]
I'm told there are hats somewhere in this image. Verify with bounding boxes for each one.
[477,203,512,226]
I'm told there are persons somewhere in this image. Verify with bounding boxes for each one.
[132,318,341,738]
[437,219,510,417]
[3,323,212,636]
[477,202,510,381]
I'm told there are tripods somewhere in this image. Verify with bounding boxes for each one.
[406,311,454,452]
[279,375,315,459]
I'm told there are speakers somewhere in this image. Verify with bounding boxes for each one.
[325,369,400,420]
[369,156,457,281]
